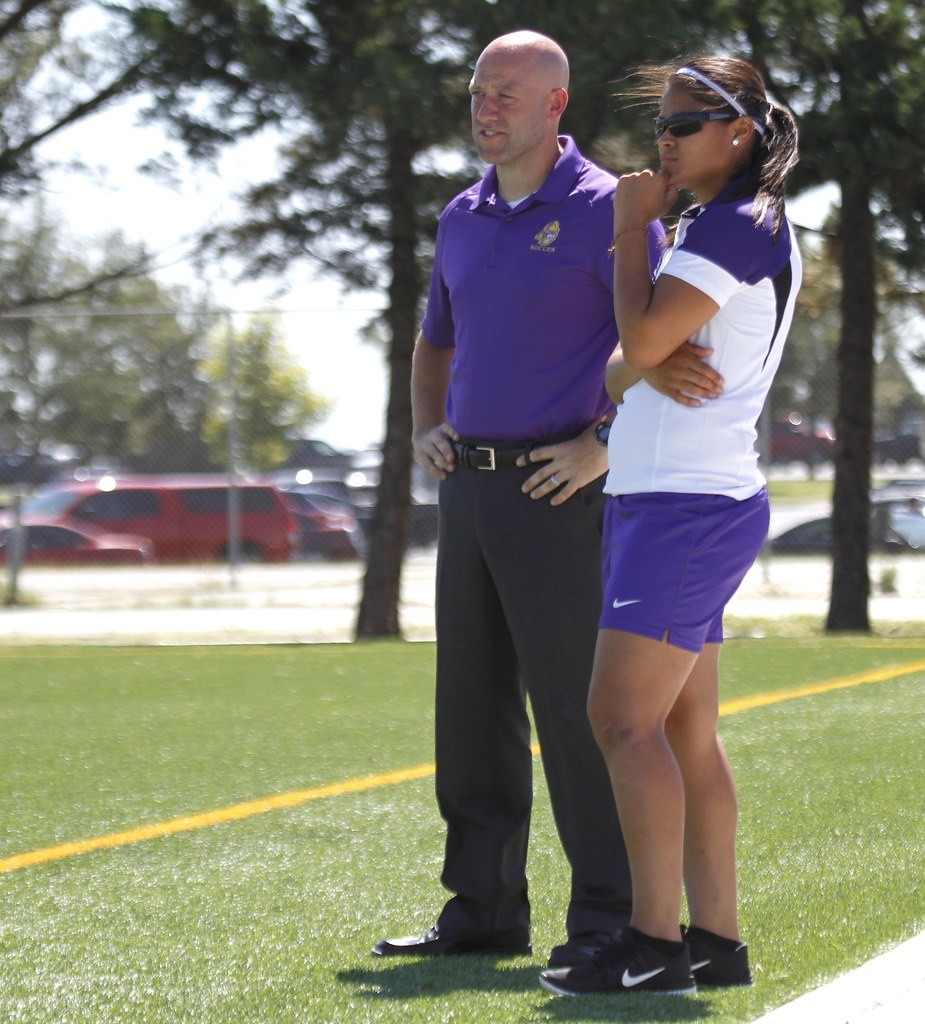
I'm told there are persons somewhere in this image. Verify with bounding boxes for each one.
[371,29,672,968]
[534,53,805,1003]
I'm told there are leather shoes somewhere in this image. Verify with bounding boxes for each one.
[547,933,620,972]
[372,926,534,962]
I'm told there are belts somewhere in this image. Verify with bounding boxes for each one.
[450,442,542,471]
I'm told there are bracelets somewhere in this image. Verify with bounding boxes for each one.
[592,417,611,447]
[606,223,649,257]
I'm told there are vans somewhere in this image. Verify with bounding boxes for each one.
[22,482,299,565]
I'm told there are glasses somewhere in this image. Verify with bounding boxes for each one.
[652,112,739,140]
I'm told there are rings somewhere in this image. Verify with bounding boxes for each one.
[550,474,561,487]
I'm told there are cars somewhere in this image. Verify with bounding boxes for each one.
[768,511,925,553]
[0,515,153,565]
[274,439,440,563]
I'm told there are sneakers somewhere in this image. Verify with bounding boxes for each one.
[539,934,699,999]
[677,923,753,992]
